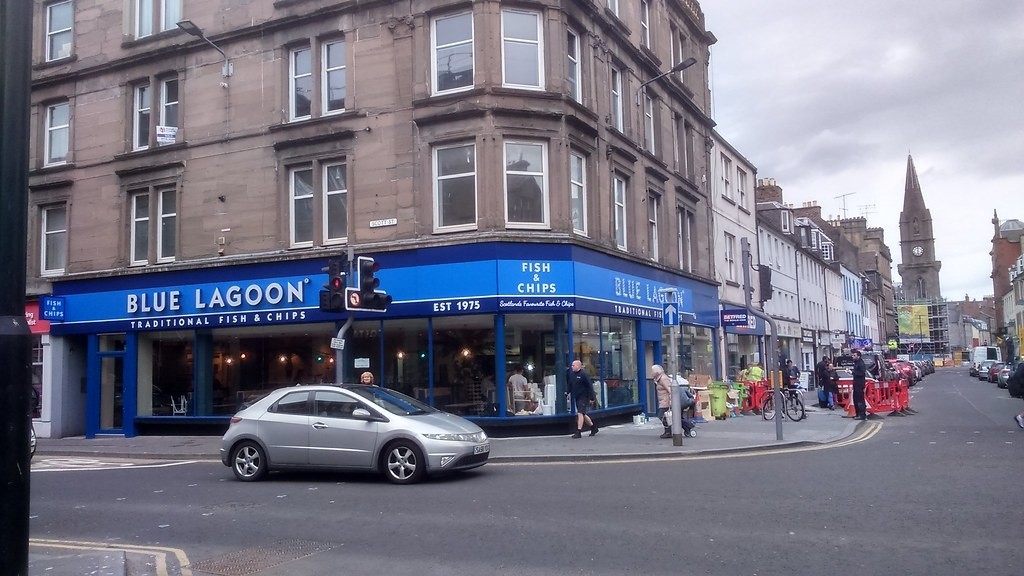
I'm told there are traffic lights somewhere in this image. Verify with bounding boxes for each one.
[328,254,347,312]
[345,255,393,312]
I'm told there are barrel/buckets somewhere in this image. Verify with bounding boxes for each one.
[632,416,645,425]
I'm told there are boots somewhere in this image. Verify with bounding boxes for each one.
[589,425,598,436]
[660,426,672,438]
[572,429,581,438]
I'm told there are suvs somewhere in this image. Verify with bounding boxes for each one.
[837,351,886,380]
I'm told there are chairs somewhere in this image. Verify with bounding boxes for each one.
[170,396,188,416]
[506,381,533,414]
[319,395,331,417]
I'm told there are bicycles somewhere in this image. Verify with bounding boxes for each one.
[763,388,804,422]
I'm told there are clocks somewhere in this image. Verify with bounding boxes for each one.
[913,246,923,256]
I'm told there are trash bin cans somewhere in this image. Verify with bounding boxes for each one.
[708,381,729,420]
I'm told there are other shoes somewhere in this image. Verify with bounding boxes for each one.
[830,406,835,410]
[852,415,865,420]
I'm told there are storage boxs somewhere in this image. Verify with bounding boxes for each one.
[689,374,710,387]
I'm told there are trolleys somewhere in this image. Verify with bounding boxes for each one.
[680,388,697,437]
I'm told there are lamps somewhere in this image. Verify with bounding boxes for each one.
[637,57,697,107]
[177,20,234,78]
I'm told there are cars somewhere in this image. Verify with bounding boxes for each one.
[969,346,1002,377]
[884,359,935,387]
[978,359,995,381]
[987,362,1006,382]
[997,364,1011,389]
[220,379,492,484]
[818,369,873,409]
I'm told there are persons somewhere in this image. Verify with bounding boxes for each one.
[847,351,866,420]
[738,362,765,415]
[361,372,385,409]
[1014,412,1024,429]
[480,373,494,402]
[506,366,529,412]
[564,360,599,438]
[815,356,839,410]
[652,365,673,438]
[783,360,800,407]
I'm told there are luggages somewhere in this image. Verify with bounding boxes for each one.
[818,384,829,408]
